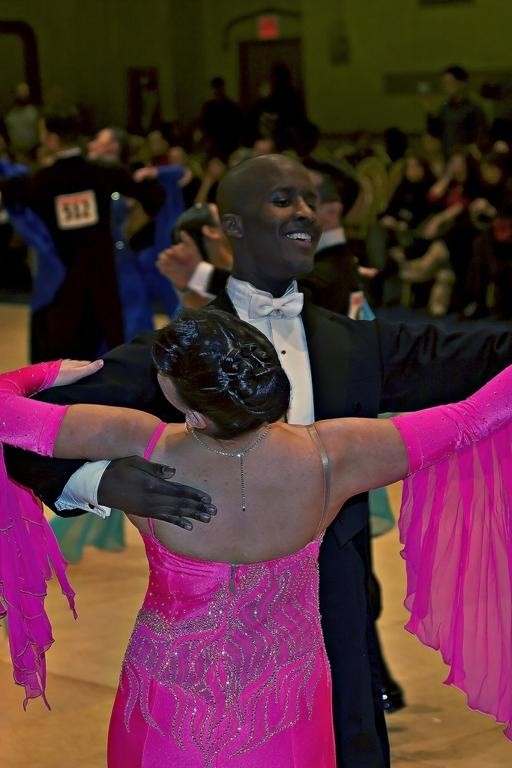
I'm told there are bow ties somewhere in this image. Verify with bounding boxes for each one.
[248,291,305,319]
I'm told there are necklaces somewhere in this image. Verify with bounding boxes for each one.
[190,421,273,512]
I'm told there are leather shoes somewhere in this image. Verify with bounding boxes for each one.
[379,678,405,712]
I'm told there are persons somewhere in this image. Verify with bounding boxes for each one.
[0,306,512,767]
[1,61,510,369]
[0,153,512,767]
